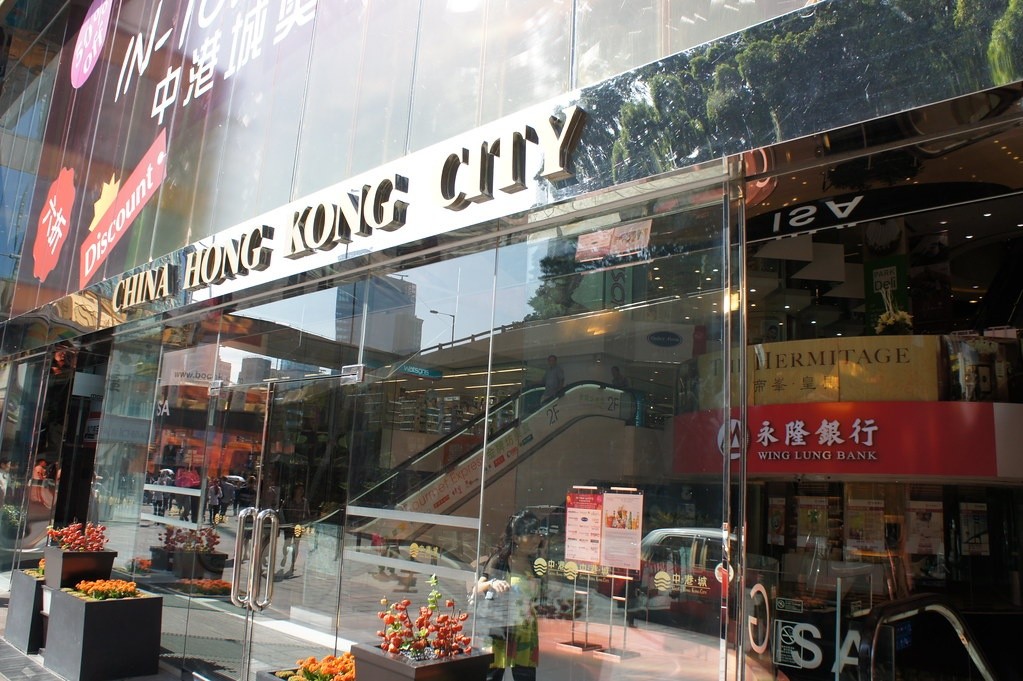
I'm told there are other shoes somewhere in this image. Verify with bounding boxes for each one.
[291,567,297,572]
[280,558,287,567]
[180,516,189,522]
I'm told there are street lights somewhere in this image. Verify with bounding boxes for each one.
[430,310,454,347]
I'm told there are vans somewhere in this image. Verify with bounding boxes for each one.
[598,526,777,642]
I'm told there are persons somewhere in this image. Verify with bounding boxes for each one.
[374,493,408,572]
[469,509,546,680]
[611,365,629,387]
[762,324,778,343]
[532,354,565,406]
[280,482,310,574]
[0,457,279,559]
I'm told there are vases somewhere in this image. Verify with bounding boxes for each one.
[150,545,228,582]
[255,667,299,681]
[43,587,163,681]
[45,545,117,589]
[4,569,46,655]
[350,638,495,681]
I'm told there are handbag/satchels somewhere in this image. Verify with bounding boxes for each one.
[466,580,506,622]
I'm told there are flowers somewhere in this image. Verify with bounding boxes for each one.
[23,558,46,579]
[125,556,153,575]
[273,652,356,681]
[44,518,109,553]
[158,524,222,552]
[376,572,472,660]
[74,578,138,601]
[170,577,233,596]
